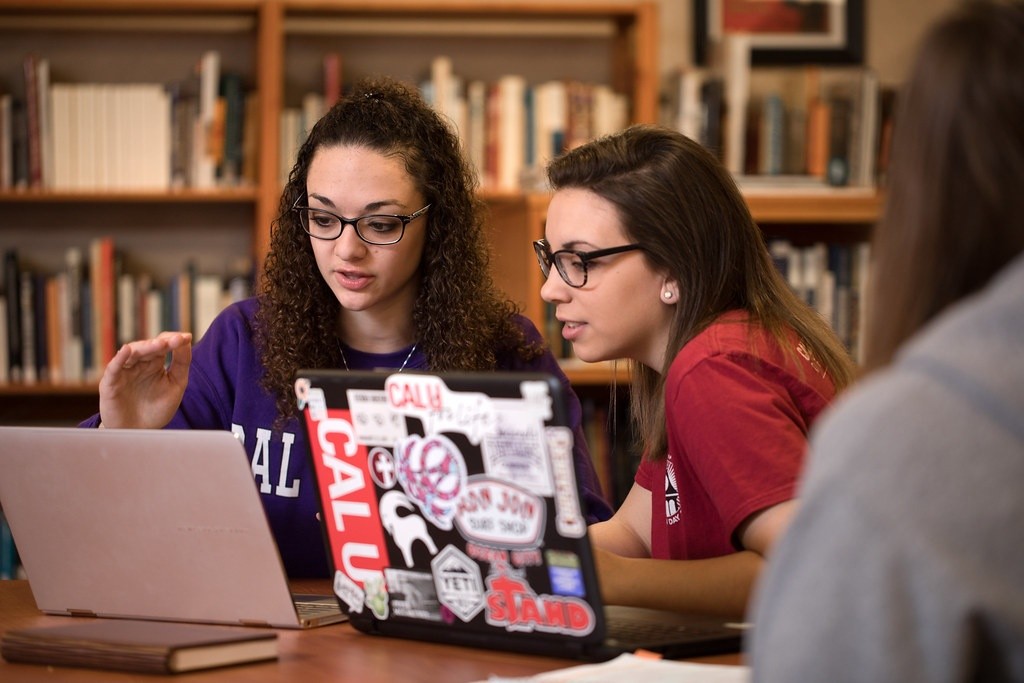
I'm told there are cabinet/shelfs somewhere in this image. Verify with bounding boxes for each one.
[0,0,906,499]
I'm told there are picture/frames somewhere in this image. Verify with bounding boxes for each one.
[691,0,866,67]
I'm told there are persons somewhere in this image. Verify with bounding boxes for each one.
[534,123,864,626]
[73,70,618,578]
[739,1,1023,683]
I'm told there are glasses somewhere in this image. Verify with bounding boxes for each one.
[532,238,643,288]
[291,191,431,245]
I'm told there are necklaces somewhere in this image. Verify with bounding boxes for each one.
[332,326,423,373]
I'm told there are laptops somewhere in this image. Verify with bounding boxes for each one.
[295,366,753,665]
[0,426,350,629]
